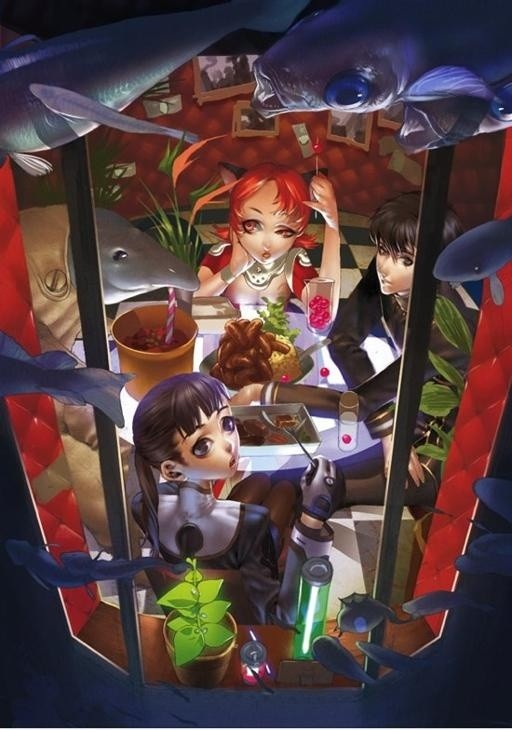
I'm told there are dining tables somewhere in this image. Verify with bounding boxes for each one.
[156,558,238,689]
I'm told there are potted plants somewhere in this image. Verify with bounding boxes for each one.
[192,56,416,153]
